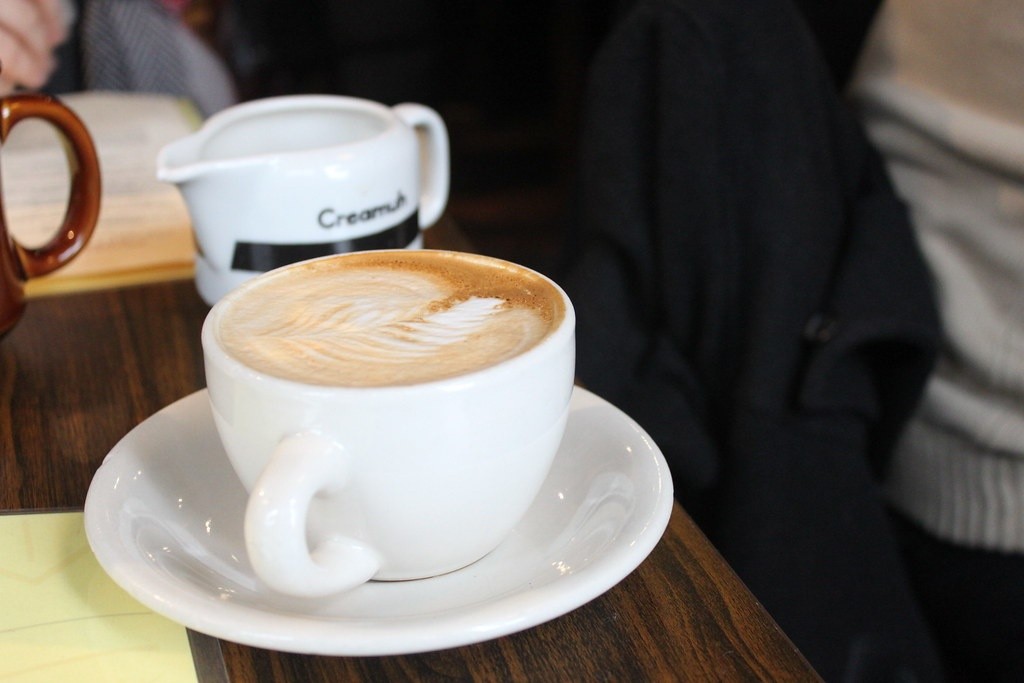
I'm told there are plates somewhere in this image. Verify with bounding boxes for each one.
[83,385,675,657]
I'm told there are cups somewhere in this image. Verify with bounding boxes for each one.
[201,249,577,596]
[156,92,449,310]
[0,94,101,332]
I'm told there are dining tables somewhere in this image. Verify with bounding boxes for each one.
[0,93,827,683]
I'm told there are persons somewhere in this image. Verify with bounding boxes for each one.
[1,0,1023,683]
